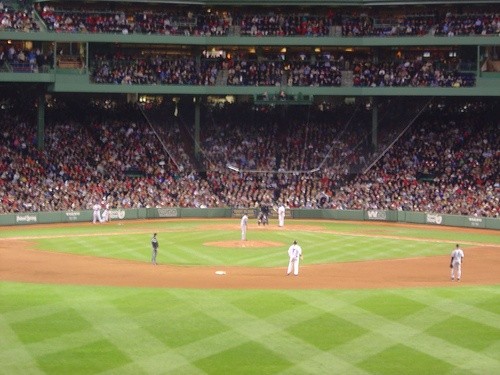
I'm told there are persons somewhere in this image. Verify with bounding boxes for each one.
[152,233,159,264]
[287,240,303,276]
[450,245,464,281]
[0,3,500,227]
[241,213,249,241]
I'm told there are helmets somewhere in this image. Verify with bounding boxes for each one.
[293,240,298,244]
[244,212,247,215]
